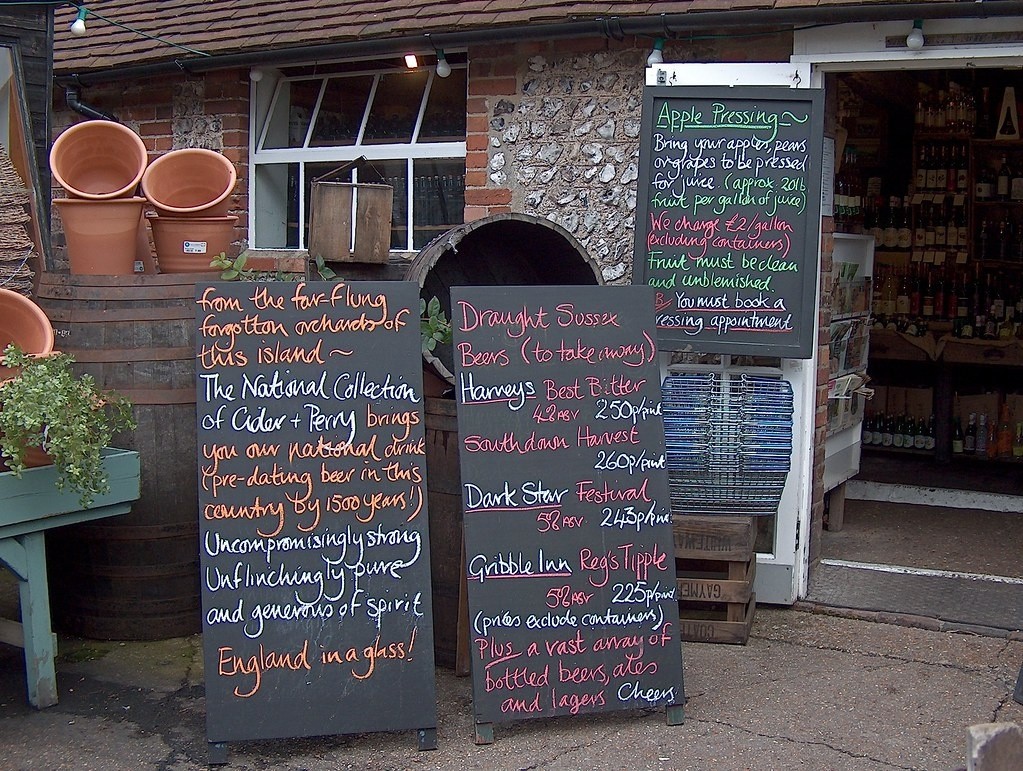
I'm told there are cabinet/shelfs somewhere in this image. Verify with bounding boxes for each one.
[292,135,465,231]
[840,134,1023,468]
[822,233,875,532]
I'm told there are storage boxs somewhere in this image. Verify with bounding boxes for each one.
[671,513,757,645]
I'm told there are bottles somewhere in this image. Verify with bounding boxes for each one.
[834,80,1023,459]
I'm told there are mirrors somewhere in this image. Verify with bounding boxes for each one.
[1,34,54,275]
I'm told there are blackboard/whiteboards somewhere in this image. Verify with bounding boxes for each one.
[631,84,826,360]
[449,285,688,725]
[191,280,441,743]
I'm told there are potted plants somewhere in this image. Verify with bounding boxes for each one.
[0,342,138,512]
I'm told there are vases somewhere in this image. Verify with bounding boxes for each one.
[148,217,236,272]
[50,118,148,199]
[141,148,236,217]
[0,289,55,394]
[53,196,157,277]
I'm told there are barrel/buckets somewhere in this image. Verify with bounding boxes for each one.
[49,119,240,276]
[37,269,241,642]
[307,175,393,266]
[0,287,55,386]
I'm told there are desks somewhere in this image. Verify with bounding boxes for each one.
[0,445,141,710]
